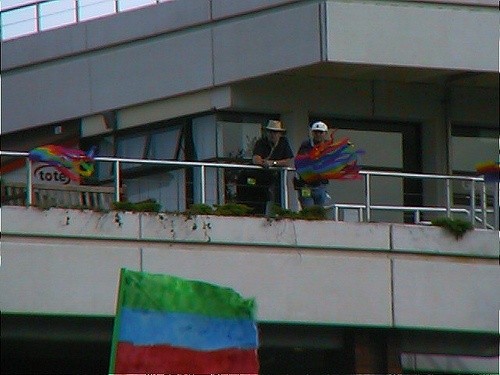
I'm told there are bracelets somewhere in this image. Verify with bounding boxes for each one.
[274,160,277,166]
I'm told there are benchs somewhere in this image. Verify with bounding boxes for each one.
[0,176,127,211]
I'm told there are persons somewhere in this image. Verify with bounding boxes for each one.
[294,121,329,210]
[252,120,296,214]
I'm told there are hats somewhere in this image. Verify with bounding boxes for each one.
[311,121,328,131]
[263,119,287,131]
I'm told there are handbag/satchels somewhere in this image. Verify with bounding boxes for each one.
[236,163,264,199]
[294,177,321,190]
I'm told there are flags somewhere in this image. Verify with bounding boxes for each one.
[108,268,260,375]
